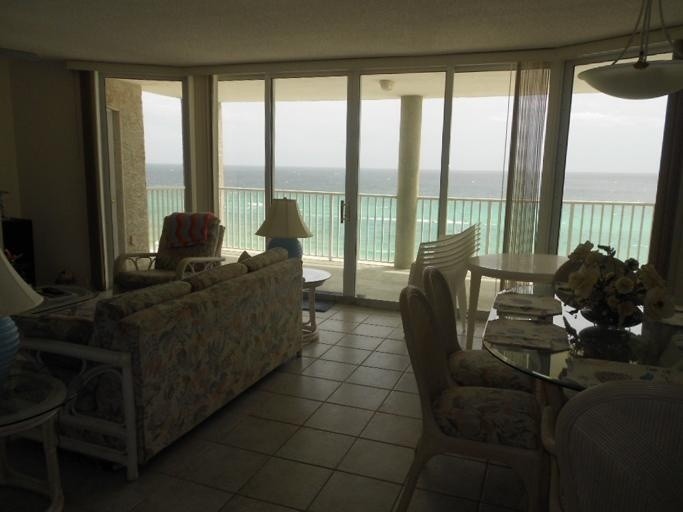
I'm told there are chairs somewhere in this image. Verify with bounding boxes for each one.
[541,380,681,511]
[111,213,226,293]
[392,265,546,511]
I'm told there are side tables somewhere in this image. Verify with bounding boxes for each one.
[303,266,332,345]
[1,367,70,512]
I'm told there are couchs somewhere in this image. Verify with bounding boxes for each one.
[12,246,303,482]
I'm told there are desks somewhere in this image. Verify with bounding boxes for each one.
[484,276,678,397]
[464,251,571,350]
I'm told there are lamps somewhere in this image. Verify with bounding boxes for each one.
[577,0,682,99]
[255,198,313,258]
[0,249,45,388]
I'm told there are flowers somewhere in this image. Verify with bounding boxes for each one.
[556,240,677,328]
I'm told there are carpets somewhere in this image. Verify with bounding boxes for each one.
[303,300,334,312]
